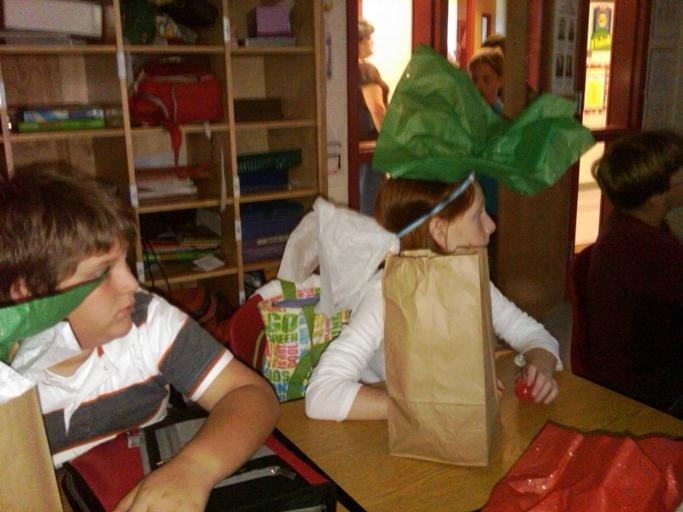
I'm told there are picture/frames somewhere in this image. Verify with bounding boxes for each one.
[539,0,585,117]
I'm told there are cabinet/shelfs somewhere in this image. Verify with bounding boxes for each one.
[0,0,328,335]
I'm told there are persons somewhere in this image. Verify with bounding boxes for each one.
[482,36,536,100]
[304,175,565,419]
[356,16,390,216]
[0,159,280,512]
[569,132,682,396]
[470,51,504,225]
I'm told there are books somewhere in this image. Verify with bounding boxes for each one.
[18,104,108,131]
[146,223,222,260]
[137,166,198,201]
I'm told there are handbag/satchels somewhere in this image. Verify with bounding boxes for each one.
[129,54,223,128]
[259,280,352,402]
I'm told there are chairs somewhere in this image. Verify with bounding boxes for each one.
[231,276,316,392]
